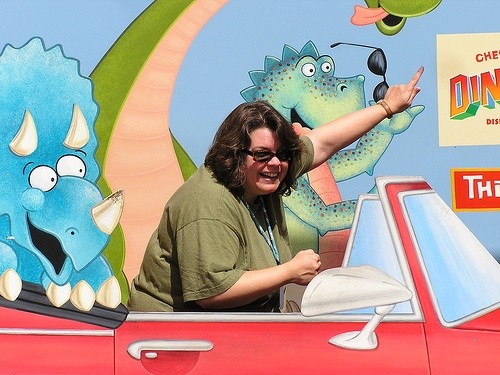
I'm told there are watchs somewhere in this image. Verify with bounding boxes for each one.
[376,99,393,119]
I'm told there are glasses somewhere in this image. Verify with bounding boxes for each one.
[221,144,296,163]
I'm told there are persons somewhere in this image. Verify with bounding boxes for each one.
[127,59,425,316]
